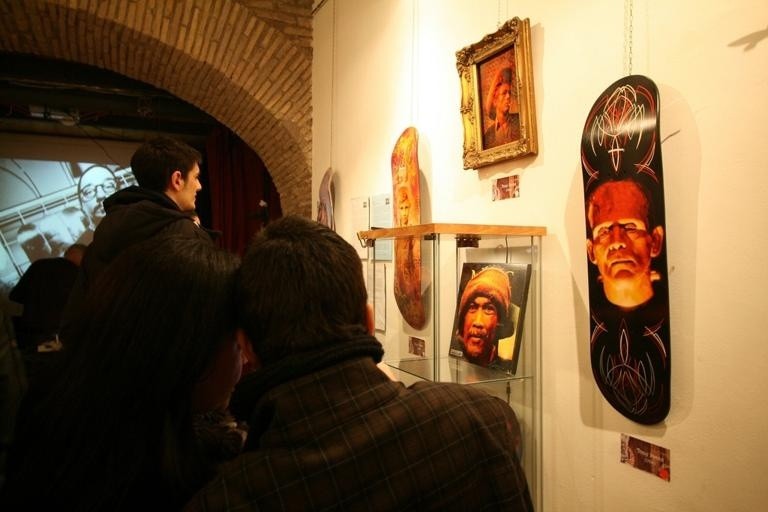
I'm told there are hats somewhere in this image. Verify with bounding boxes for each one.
[458,266,511,337]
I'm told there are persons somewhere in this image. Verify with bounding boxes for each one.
[482,66,520,148]
[1,229,251,510]
[182,210,539,510]
[79,164,121,248]
[33,134,204,365]
[456,266,515,367]
[8,242,86,378]
[395,183,416,277]
[583,171,666,335]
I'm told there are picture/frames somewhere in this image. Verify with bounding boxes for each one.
[455,15,539,170]
